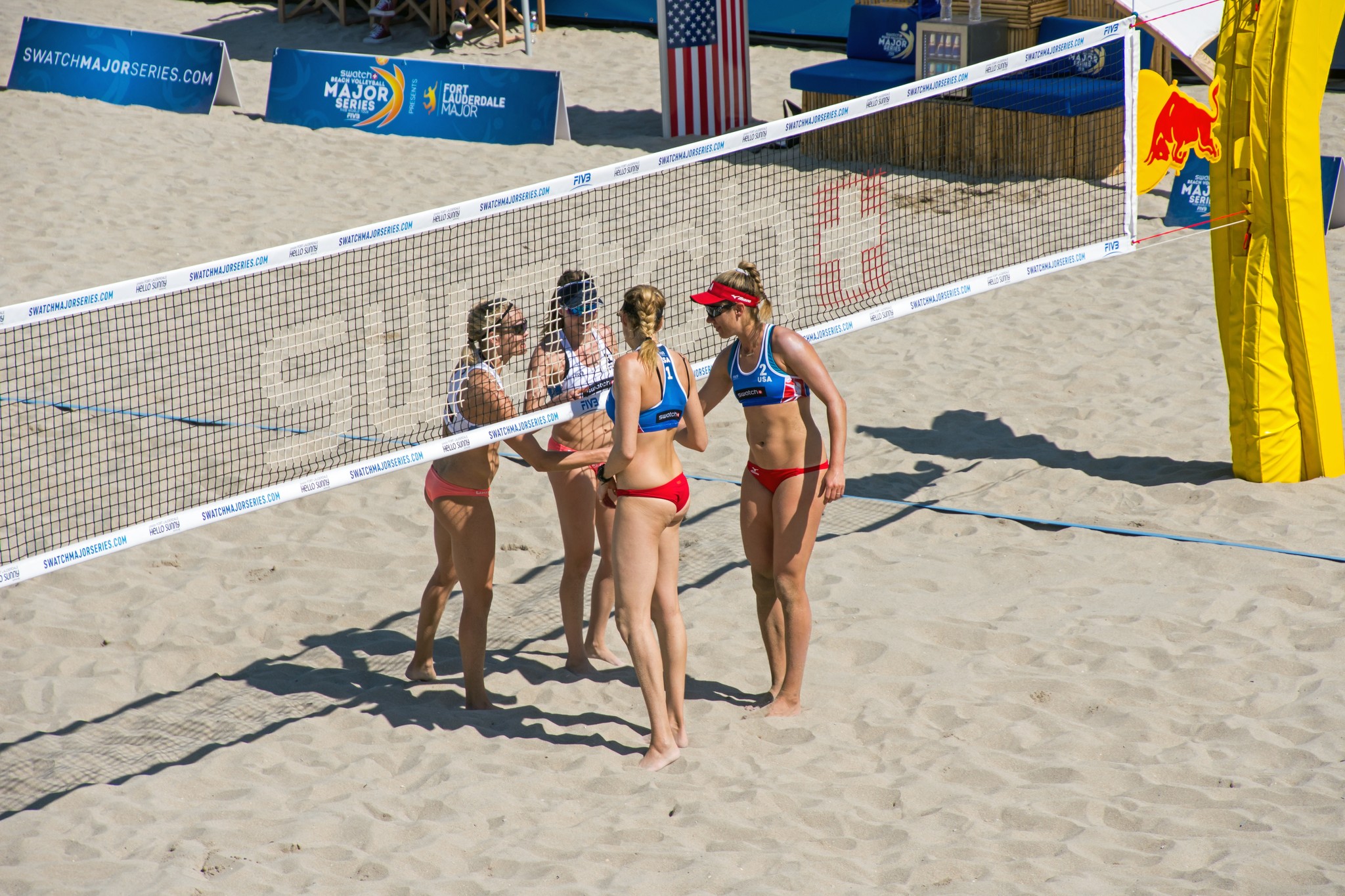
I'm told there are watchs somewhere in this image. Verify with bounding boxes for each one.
[595,464,612,486]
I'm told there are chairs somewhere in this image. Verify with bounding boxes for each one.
[789,4,927,97]
[277,0,545,48]
[971,16,1154,118]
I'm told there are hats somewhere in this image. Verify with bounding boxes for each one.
[616,299,663,319]
[557,281,604,308]
[690,280,761,308]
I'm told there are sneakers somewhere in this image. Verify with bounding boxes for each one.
[363,22,392,43]
[367,0,396,17]
[427,31,466,50]
[449,8,472,34]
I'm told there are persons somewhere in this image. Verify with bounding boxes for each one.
[698,258,848,715]
[522,270,620,683]
[406,296,613,711]
[595,284,709,788]
[361,0,395,46]
[427,0,472,52]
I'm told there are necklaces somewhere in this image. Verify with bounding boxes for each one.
[738,350,755,357]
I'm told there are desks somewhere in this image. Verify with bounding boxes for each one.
[916,15,1008,98]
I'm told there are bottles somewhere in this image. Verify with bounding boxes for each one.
[939,0,952,21]
[969,0,982,22]
[530,11,538,43]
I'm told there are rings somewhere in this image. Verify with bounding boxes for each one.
[598,500,603,502]
[612,489,617,491]
[836,493,841,494]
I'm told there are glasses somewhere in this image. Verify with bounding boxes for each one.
[705,303,736,318]
[491,319,527,335]
[553,297,597,316]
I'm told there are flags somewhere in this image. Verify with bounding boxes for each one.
[664,1,750,137]
